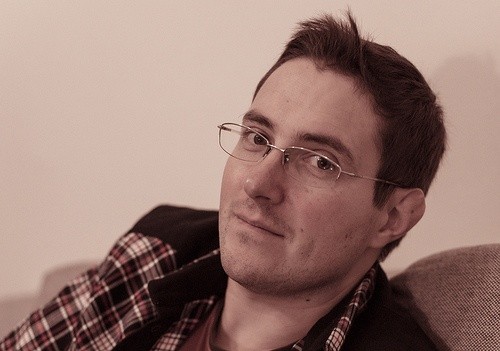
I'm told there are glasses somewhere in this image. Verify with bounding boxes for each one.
[216,121,405,189]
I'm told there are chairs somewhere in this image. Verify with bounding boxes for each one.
[405,243,500,351]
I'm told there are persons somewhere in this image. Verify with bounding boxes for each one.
[0,8,447,351]
